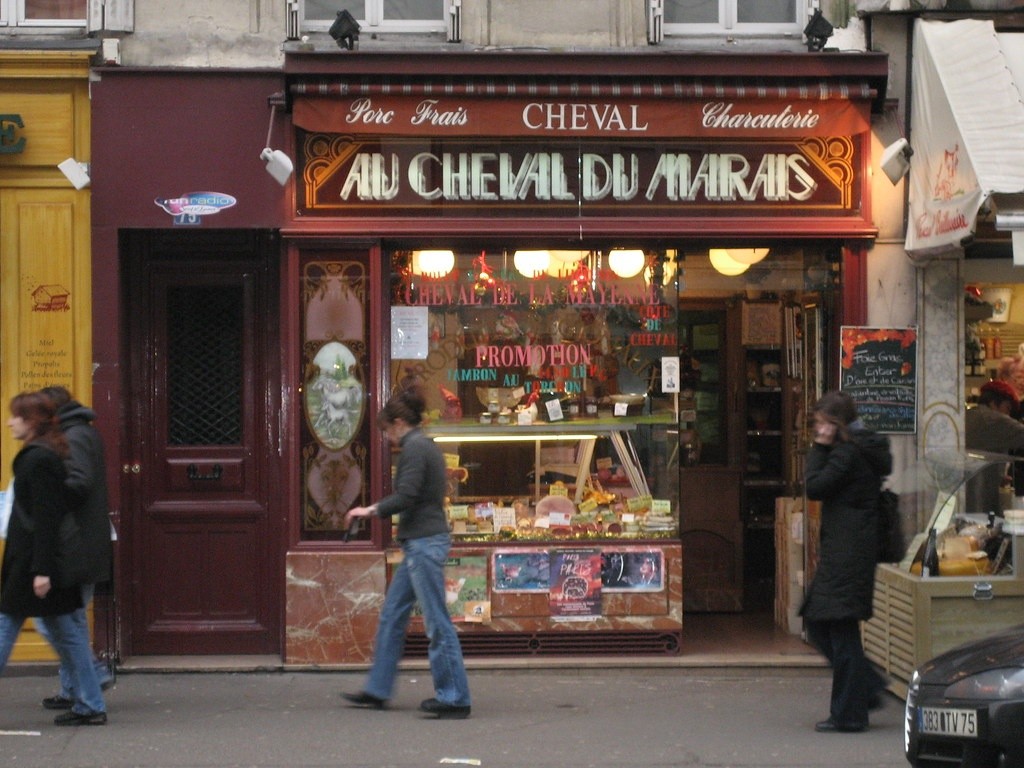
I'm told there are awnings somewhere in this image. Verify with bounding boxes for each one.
[287,75,879,139]
[905,17,1024,263]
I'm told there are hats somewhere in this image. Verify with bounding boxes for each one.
[980,381,1021,411]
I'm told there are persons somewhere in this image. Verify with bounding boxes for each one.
[340,384,471,720]
[965,378,1024,519]
[0,386,114,728]
[679,429,722,465]
[794,390,892,733]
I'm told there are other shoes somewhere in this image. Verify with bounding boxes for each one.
[867,691,890,711]
[814,719,869,732]
[421,699,470,718]
[339,692,384,709]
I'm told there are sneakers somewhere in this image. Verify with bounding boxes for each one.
[53,708,107,725]
[42,696,72,710]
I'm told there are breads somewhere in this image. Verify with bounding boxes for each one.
[911,536,988,578]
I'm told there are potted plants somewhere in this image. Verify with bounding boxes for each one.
[742,262,772,301]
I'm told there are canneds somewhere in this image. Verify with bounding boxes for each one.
[479,400,511,424]
[566,395,598,418]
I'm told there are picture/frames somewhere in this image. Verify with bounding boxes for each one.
[803,303,824,433]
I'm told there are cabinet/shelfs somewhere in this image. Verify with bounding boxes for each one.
[744,342,788,534]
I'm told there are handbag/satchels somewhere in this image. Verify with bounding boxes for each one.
[876,489,908,565]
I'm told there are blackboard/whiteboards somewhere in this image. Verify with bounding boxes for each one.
[840,328,917,433]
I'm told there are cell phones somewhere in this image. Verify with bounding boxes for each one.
[829,420,840,435]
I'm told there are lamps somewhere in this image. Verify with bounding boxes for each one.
[548,250,589,262]
[802,9,835,52]
[328,8,361,50]
[708,249,750,277]
[513,251,550,279]
[727,248,770,264]
[544,260,579,279]
[258,93,293,186]
[608,250,645,278]
[418,250,455,278]
[56,158,91,191]
[879,97,913,186]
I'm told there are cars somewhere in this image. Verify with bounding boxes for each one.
[904,623,1024,768]
